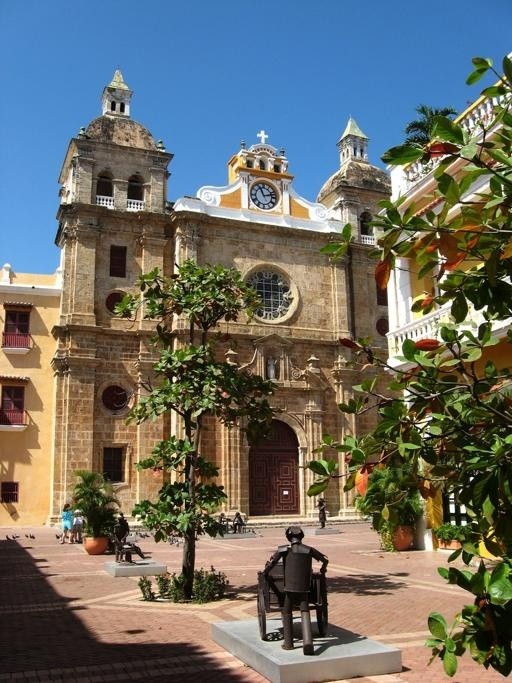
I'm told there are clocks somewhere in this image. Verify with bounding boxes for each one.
[249,177,282,212]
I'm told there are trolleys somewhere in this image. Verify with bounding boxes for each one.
[257,560,328,640]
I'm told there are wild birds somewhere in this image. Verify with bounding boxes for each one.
[248,528,264,536]
[5,532,37,542]
[131,530,153,538]
[55,533,61,540]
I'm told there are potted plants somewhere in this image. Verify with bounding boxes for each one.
[355,469,423,553]
[65,469,121,555]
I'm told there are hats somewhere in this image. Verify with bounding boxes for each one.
[317,498,325,502]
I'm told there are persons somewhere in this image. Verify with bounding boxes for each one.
[112,516,130,561]
[217,512,231,535]
[72,509,85,543]
[233,512,246,534]
[261,524,329,654]
[317,497,327,528]
[60,503,74,543]
